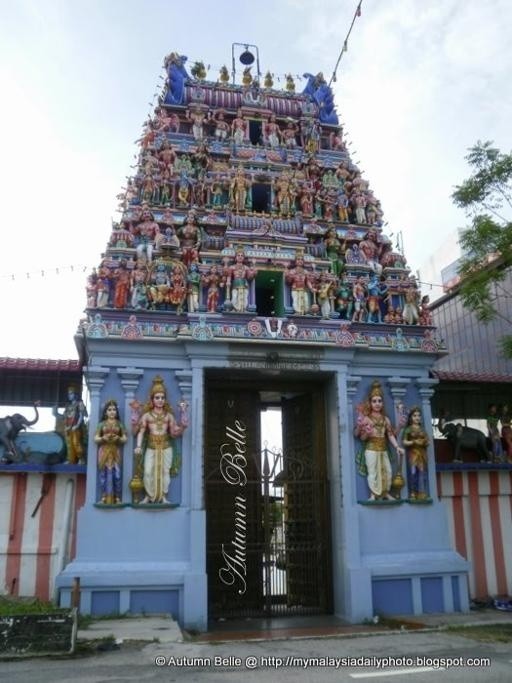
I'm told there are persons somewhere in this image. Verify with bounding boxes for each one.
[53,385,85,465]
[499,403,511,463]
[92,398,127,505]
[85,54,435,329]
[399,407,433,501]
[487,403,505,463]
[353,385,406,501]
[129,376,189,502]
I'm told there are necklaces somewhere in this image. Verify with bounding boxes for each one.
[106,420,117,426]
[368,413,387,431]
[148,408,169,423]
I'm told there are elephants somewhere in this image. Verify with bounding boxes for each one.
[0,400,41,459]
[437,408,491,463]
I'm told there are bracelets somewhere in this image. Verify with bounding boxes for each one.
[410,425,421,430]
[395,445,399,449]
[413,439,416,445]
[117,437,120,443]
[102,437,107,443]
